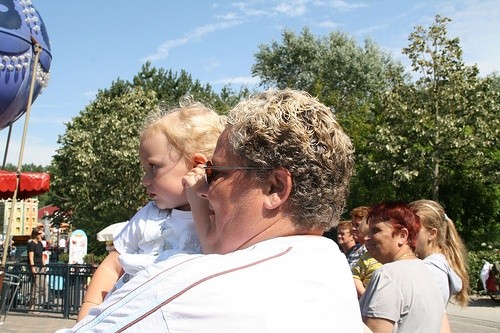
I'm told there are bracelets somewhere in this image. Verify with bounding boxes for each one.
[85,300,100,306]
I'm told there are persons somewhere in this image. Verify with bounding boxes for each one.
[76,102,227,325]
[336,207,386,295]
[27,227,44,314]
[55,90,363,333]
[360,200,450,333]
[408,199,469,308]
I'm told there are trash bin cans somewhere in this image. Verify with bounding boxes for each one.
[0,262,31,308]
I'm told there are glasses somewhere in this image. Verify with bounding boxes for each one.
[203,160,274,184]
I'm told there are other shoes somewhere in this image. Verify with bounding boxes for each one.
[31,305,42,309]
